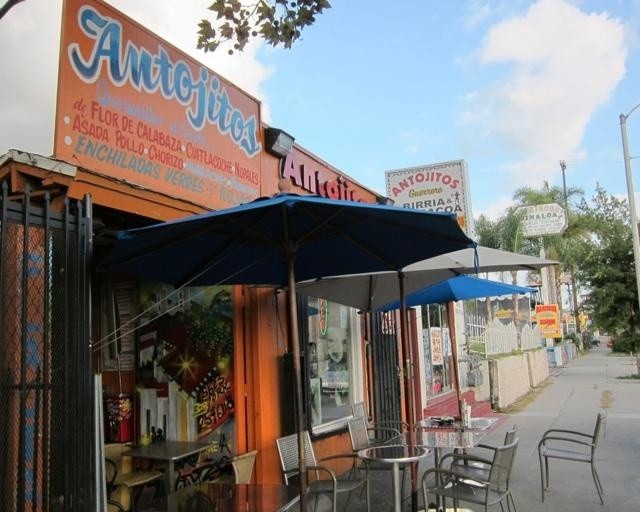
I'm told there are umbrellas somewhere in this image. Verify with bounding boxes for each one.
[357,274,539,468]
[244,243,562,512]
[111,173,478,511]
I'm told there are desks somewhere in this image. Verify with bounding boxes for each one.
[122,440,212,512]
[139,483,309,512]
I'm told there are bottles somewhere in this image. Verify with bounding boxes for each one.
[461,402,471,427]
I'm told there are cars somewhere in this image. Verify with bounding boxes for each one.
[590,335,601,346]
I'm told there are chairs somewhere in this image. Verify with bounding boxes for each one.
[104,443,162,511]
[192,449,261,485]
[273,401,519,512]
[537,412,605,506]
